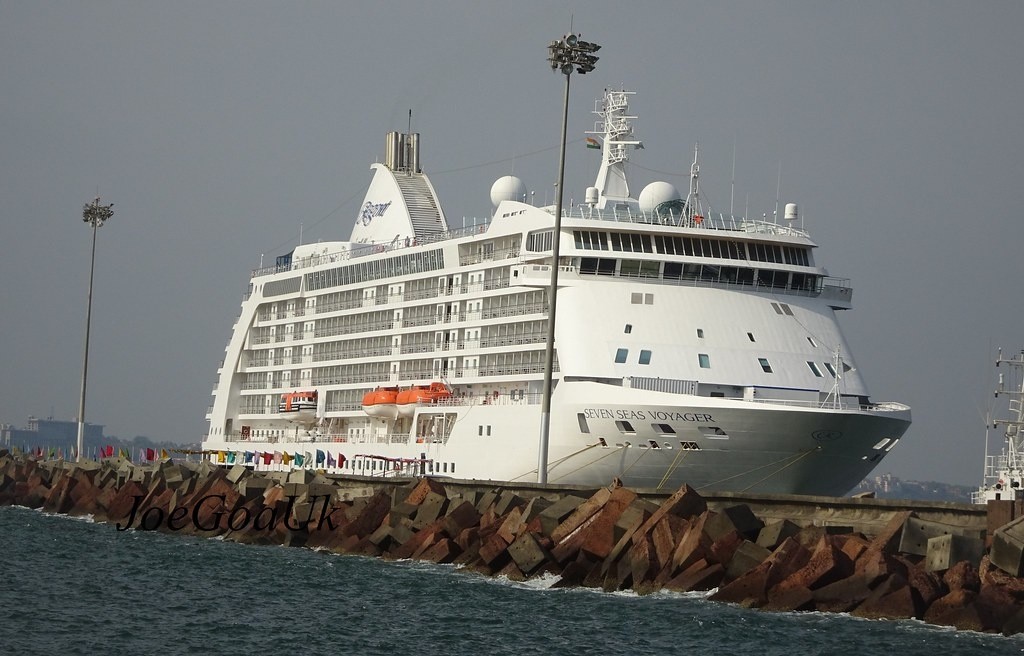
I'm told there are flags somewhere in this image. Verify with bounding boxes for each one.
[245,451,254,462]
[236,451,245,465]
[11,445,113,463]
[146,447,154,460]
[264,452,272,464]
[585,137,601,150]
[228,450,236,464]
[338,453,346,468]
[328,451,336,466]
[254,451,261,465]
[162,448,168,459]
[295,451,303,467]
[316,450,326,463]
[119,447,124,457]
[218,451,227,462]
[305,451,313,467]
[154,448,159,461]
[140,448,146,460]
[124,447,129,457]
[284,451,291,465]
[273,450,282,463]
